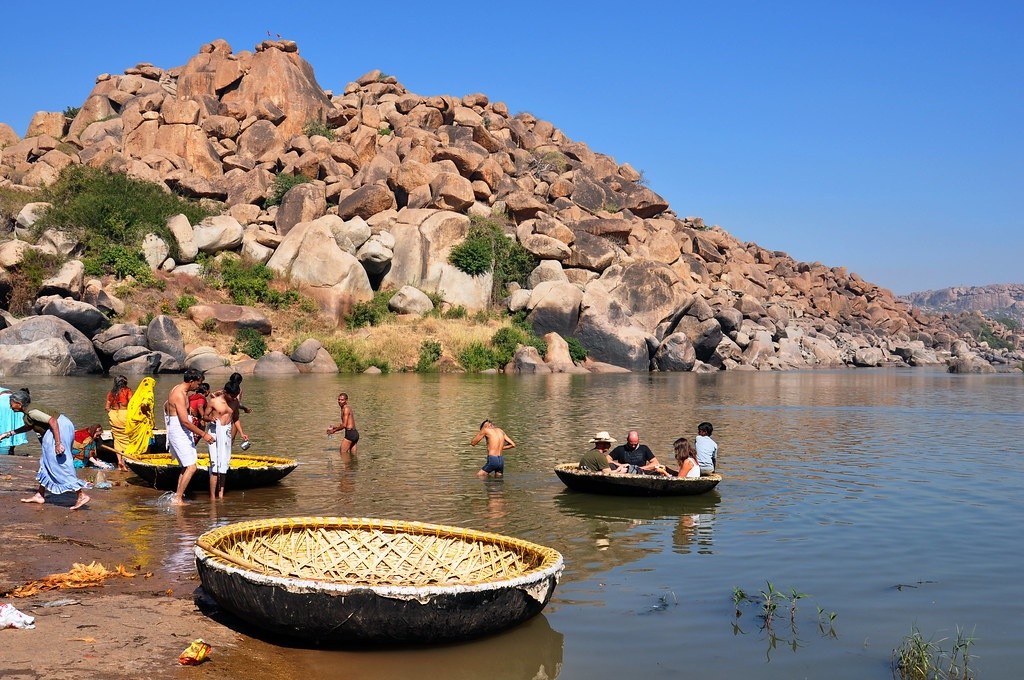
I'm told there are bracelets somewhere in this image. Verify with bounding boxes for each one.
[54,441,62,447]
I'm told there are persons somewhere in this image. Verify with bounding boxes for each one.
[471,419,516,476]
[577,430,660,474]
[655,438,700,478]
[695,422,717,474]
[72,424,109,470]
[327,393,359,453]
[105,375,155,473]
[0,391,91,510]
[163,368,253,499]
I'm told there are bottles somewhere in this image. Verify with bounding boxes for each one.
[328,424,332,438]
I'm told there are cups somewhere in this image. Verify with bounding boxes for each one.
[239,440,251,450]
